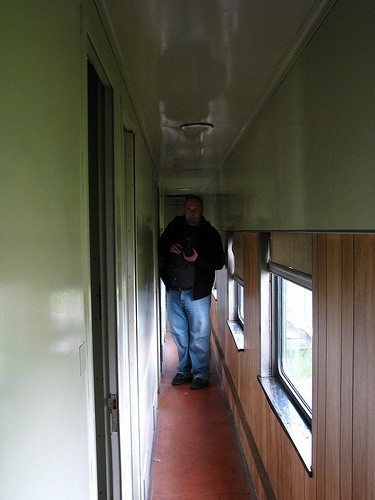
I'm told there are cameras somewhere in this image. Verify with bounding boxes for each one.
[178,240,198,257]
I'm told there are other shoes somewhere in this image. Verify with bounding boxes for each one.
[172,374,193,385]
[190,379,209,390]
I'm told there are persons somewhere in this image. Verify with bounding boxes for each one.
[158,194,224,389]
